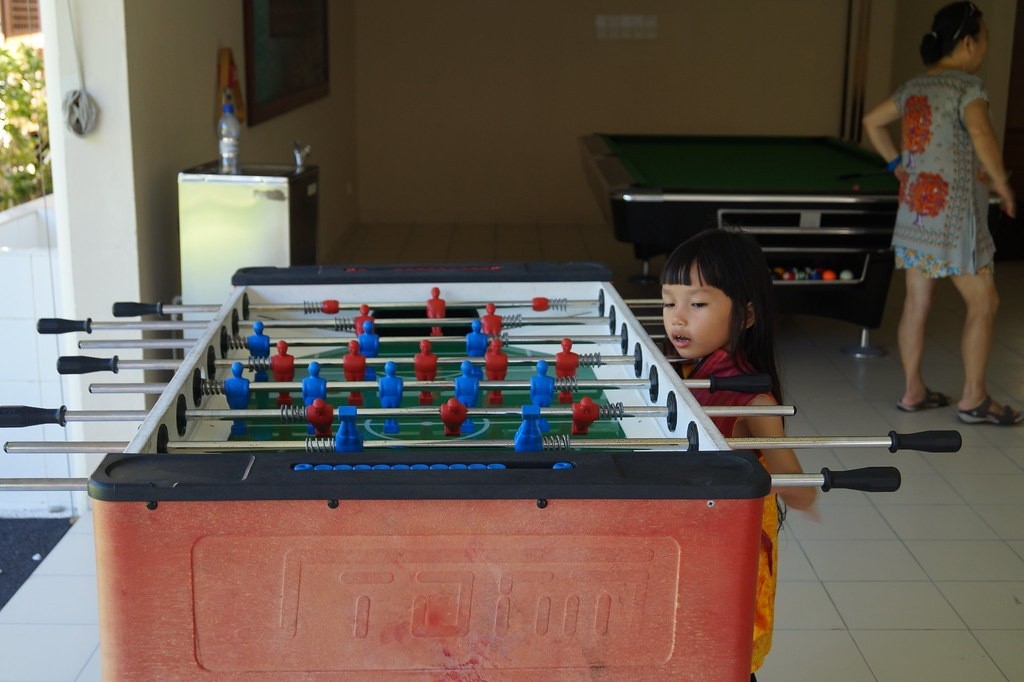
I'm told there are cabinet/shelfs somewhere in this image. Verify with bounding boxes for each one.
[176,159,321,358]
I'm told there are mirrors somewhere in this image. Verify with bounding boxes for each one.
[242,0,329,127]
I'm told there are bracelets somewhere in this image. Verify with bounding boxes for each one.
[886,156,902,173]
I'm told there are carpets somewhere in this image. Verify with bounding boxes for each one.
[0,517,79,611]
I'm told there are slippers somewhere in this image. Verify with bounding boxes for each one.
[897,386,952,412]
[956,395,1024,424]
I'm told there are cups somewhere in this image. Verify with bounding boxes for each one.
[291,143,312,166]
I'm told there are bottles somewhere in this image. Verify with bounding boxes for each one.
[218,105,242,175]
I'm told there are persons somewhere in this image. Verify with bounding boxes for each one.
[860,1,1024,426]
[660,229,816,682]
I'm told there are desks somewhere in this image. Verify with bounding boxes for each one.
[578,132,900,358]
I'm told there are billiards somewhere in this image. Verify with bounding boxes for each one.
[770,265,854,281]
